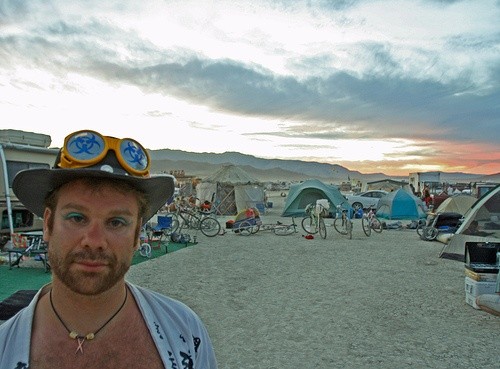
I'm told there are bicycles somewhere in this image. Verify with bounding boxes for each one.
[163,197,221,238]
[255,217,299,236]
[361,204,383,237]
[416,208,443,241]
[333,202,353,239]
[301,203,327,239]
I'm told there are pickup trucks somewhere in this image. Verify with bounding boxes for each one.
[415,192,453,209]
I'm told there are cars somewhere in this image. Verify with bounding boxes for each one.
[347,190,389,212]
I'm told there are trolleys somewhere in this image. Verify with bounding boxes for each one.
[224,215,262,236]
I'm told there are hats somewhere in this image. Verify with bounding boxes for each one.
[11,136,176,227]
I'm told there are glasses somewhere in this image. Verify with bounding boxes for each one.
[58,129,151,177]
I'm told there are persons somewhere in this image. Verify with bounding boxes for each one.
[447,184,453,195]
[170,195,212,212]
[0,130,219,369]
[423,186,432,209]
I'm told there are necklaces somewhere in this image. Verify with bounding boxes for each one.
[49,284,128,354]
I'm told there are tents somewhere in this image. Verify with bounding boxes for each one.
[196,163,500,264]
